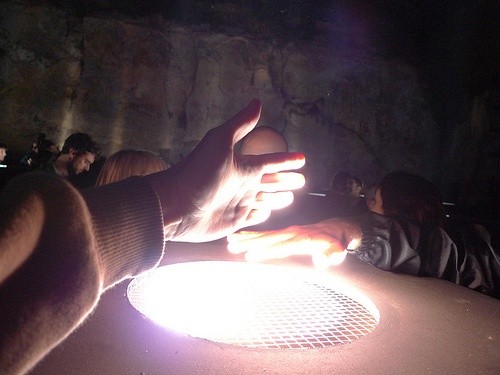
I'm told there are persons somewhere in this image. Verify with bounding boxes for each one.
[0,96,499,374]
